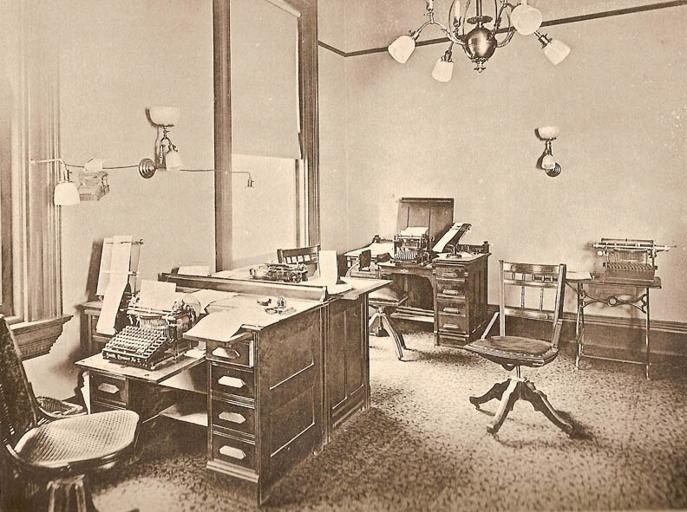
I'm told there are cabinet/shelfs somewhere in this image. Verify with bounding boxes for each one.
[213,264,396,443]
[345,196,494,350]
[72,277,324,509]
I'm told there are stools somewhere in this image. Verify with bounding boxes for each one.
[365,288,410,360]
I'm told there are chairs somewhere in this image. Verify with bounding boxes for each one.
[278,244,322,264]
[0,313,142,512]
[463,259,582,440]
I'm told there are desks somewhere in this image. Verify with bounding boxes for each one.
[562,273,663,383]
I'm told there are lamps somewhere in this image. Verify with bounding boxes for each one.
[137,105,180,181]
[537,121,562,178]
[387,0,570,82]
[26,157,81,208]
